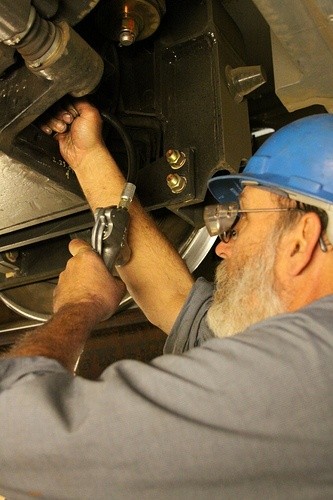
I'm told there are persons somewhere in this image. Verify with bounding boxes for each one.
[0,96,333,500]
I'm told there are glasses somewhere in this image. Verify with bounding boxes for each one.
[203,202,328,253]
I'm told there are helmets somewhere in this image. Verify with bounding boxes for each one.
[206,113,333,245]
